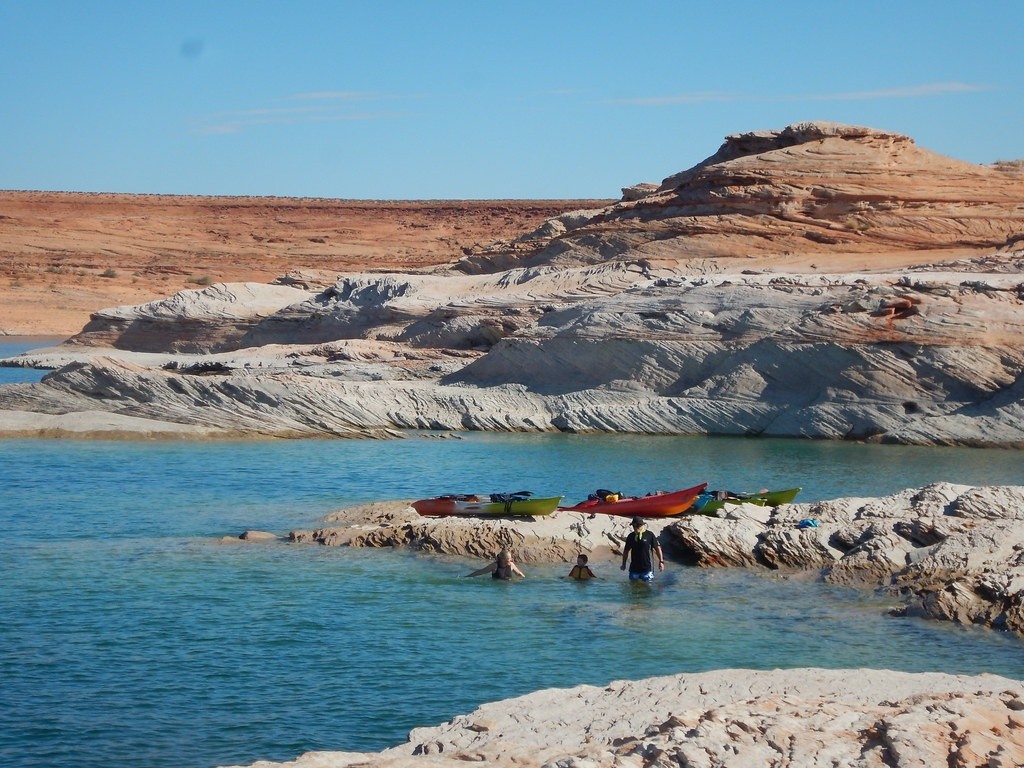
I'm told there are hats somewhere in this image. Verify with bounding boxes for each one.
[630,516,646,525]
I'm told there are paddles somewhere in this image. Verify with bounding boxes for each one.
[440,491,535,499]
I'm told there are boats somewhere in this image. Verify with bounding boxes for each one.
[558,482,709,517]
[646,487,802,517]
[411,491,566,516]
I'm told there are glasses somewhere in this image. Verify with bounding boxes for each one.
[633,525,641,528]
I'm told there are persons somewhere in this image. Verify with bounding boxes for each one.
[467,550,525,579]
[569,554,596,578]
[620,516,664,582]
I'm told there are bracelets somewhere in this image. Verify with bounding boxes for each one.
[660,561,664,563]
